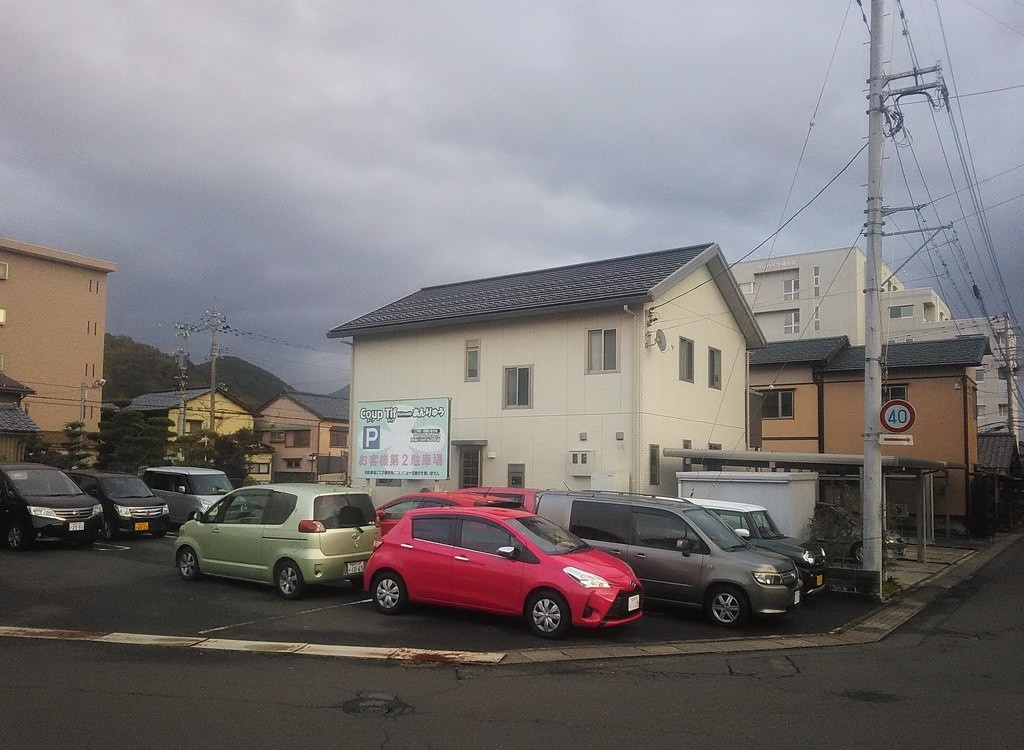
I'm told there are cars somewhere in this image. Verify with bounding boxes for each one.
[171,481,382,602]
[362,506,646,641]
[813,499,909,568]
[375,485,540,540]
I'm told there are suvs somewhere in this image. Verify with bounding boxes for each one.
[635,494,828,601]
[143,466,248,526]
[0,462,104,552]
[57,469,171,542]
[523,487,804,630]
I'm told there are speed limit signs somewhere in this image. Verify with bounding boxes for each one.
[879,398,917,433]
